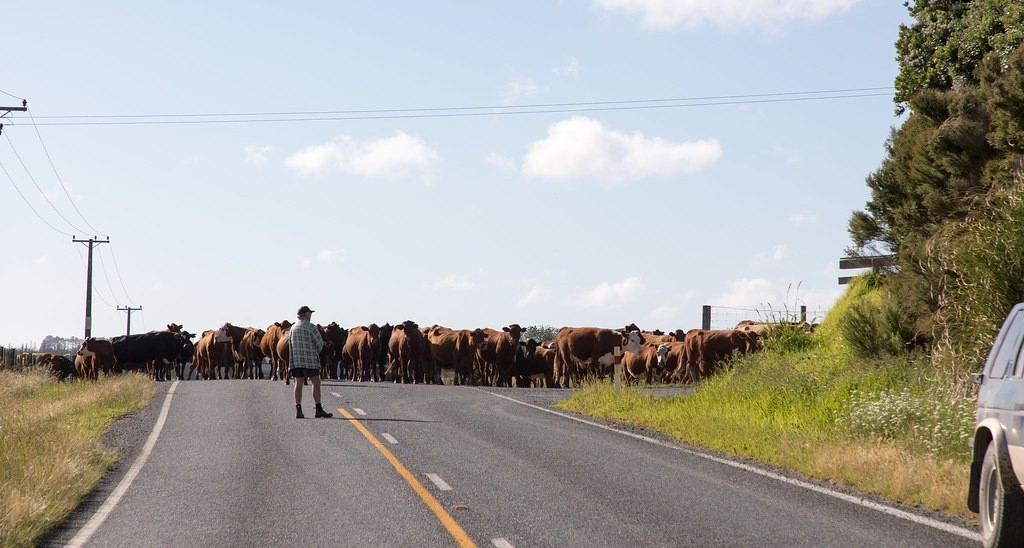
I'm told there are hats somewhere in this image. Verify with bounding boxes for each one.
[297,306,315,315]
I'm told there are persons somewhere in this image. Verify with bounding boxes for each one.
[287,306,333,418]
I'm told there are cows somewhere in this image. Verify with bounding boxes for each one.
[15,319,815,389]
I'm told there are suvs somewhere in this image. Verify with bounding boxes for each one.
[966,299,1024,548]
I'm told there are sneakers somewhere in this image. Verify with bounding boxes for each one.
[315,407,332,418]
[296,409,304,418]
